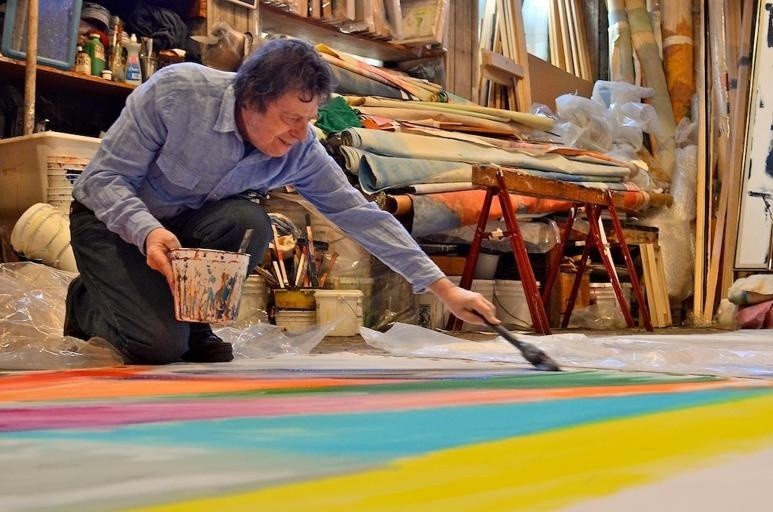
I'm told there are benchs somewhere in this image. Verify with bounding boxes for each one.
[446,163,654,335]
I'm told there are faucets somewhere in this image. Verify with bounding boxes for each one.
[33,119,49,133]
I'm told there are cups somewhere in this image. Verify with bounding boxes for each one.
[74,53,91,76]
[140,56,158,84]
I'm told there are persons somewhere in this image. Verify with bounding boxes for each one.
[62,36,502,366]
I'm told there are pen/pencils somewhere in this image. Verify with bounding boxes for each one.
[253,215,339,293]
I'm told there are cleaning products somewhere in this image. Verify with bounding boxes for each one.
[73,16,143,86]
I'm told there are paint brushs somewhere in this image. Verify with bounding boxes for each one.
[470,309,560,373]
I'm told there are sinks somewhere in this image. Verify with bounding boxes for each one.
[0,130,102,209]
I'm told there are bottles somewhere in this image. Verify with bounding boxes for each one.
[86,22,142,85]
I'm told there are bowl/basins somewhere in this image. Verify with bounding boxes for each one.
[428,254,466,276]
[275,311,315,330]
[273,289,315,310]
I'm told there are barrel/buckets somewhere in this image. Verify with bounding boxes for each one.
[10,154,635,338]
[553,261,588,333]
[443,278,495,334]
[588,281,632,333]
[497,278,544,334]
[169,248,254,329]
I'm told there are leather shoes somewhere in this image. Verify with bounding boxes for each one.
[63,277,90,341]
[182,330,234,362]
